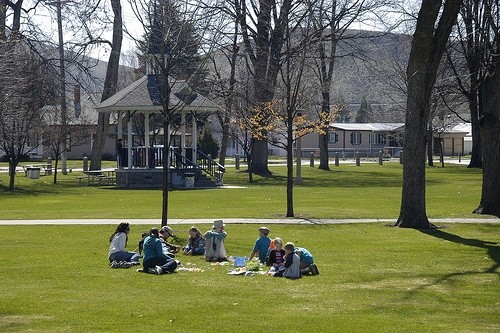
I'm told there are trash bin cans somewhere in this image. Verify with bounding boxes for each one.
[27,168,40,179]
[214,170,223,185]
[184,172,195,187]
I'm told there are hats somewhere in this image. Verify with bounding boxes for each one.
[258,227,269,234]
[270,239,275,249]
[161,226,174,237]
[212,220,226,228]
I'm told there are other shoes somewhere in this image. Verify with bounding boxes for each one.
[148,268,155,274]
[309,263,319,275]
[155,265,162,275]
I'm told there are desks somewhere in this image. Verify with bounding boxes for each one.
[82,168,117,186]
[32,164,52,176]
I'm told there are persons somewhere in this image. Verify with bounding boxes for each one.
[108,222,140,267]
[249,226,320,278]
[138,220,228,275]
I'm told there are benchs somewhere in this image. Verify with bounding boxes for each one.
[77,175,117,179]
[41,167,56,172]
